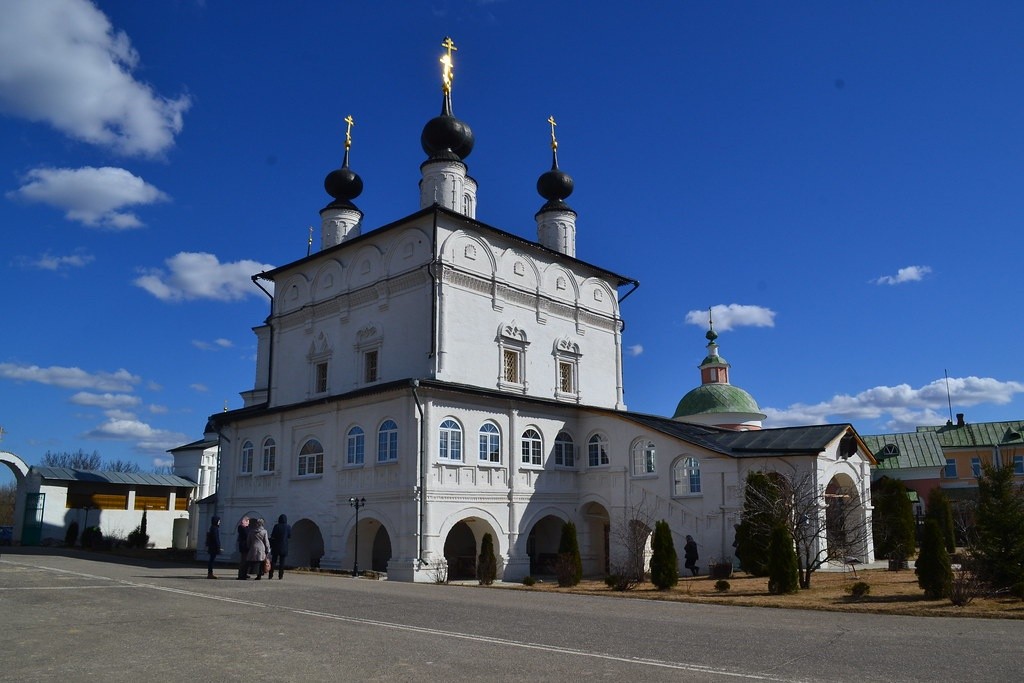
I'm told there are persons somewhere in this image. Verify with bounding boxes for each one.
[204,516,224,580]
[246,518,270,580]
[268,514,292,580]
[684,535,700,576]
[236,515,252,580]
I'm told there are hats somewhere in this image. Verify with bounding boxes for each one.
[243,516,249,520]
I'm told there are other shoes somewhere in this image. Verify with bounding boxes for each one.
[255,577,261,580]
[246,575,250,578]
[238,576,246,580]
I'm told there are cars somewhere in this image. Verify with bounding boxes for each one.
[0,527,12,545]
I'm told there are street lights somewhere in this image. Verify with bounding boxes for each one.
[350,496,366,577]
[80,507,94,528]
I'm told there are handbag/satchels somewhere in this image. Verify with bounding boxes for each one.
[205,531,214,546]
[262,554,272,573]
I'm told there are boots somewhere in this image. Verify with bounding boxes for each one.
[207,571,217,579]
